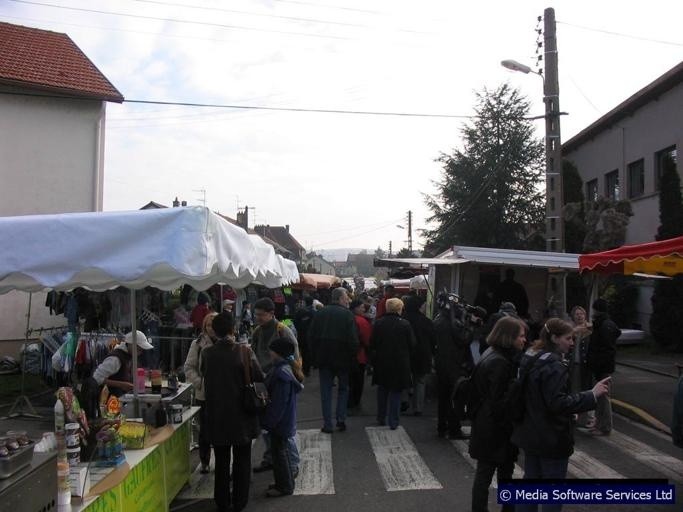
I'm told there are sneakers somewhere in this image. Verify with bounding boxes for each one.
[266,487,284,498]
[592,428,611,436]
[253,463,271,472]
[449,432,471,440]
[200,464,210,474]
[321,427,333,433]
[400,402,408,412]
[336,422,346,431]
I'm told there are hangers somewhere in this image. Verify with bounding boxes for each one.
[138,308,195,331]
[25,325,125,345]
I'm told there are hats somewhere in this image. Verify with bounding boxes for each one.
[384,298,404,314]
[592,297,609,312]
[126,330,154,350]
[198,291,211,304]
[224,299,235,308]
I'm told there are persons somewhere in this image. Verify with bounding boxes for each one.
[494,314,613,510]
[169,268,621,438]
[466,315,528,511]
[199,310,271,512]
[264,337,307,498]
[248,296,305,473]
[91,329,155,406]
[184,311,223,473]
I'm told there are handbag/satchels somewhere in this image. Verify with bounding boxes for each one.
[242,382,272,412]
[493,378,525,422]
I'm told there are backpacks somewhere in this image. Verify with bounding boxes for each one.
[449,351,495,420]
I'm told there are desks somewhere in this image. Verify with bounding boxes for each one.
[56,404,203,512]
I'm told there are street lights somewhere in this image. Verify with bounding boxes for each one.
[500,60,557,316]
[396,225,411,253]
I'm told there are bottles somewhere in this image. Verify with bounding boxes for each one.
[136,368,145,395]
[172,404,182,424]
[150,369,161,395]
[64,447,81,469]
[64,422,80,450]
[54,462,72,506]
